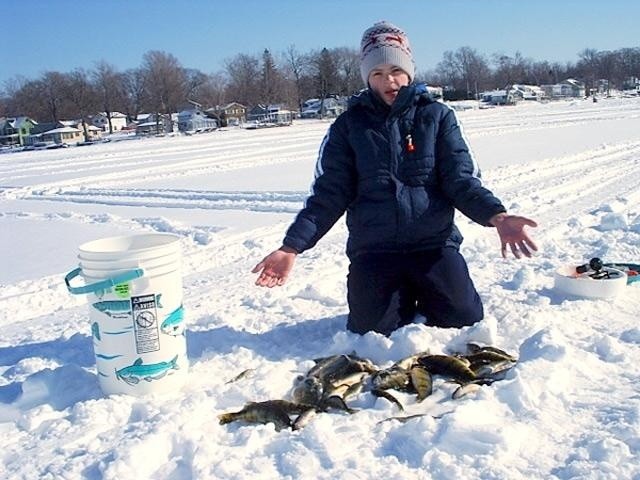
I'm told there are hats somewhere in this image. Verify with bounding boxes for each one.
[359,21,416,88]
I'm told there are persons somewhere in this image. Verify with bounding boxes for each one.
[251,21,538,336]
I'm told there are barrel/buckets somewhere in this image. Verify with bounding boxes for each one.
[64,233,187,399]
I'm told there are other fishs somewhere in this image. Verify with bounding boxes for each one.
[215,341,522,434]
